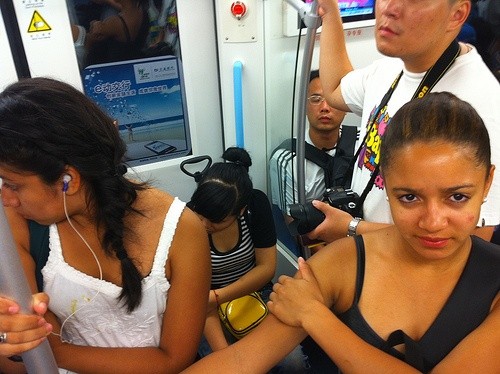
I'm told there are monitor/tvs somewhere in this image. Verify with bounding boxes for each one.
[283,0,378,37]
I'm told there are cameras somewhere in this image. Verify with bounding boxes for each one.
[288,186,364,234]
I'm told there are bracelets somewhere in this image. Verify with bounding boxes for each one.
[346,217,363,237]
[213,289,220,311]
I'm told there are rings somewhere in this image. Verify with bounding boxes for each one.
[0,332,7,344]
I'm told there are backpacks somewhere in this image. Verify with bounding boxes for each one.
[269,124,358,190]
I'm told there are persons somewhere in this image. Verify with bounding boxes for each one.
[179,93,500,374]
[268,70,361,254]
[190,147,276,353]
[306,1,500,244]
[0,78,211,374]
[65,1,182,72]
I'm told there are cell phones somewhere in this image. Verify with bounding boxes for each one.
[144,141,176,154]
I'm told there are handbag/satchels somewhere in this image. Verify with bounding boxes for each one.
[218,290,268,340]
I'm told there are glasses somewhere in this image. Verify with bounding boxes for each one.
[306,95,327,106]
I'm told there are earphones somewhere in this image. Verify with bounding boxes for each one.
[62,175,72,191]
